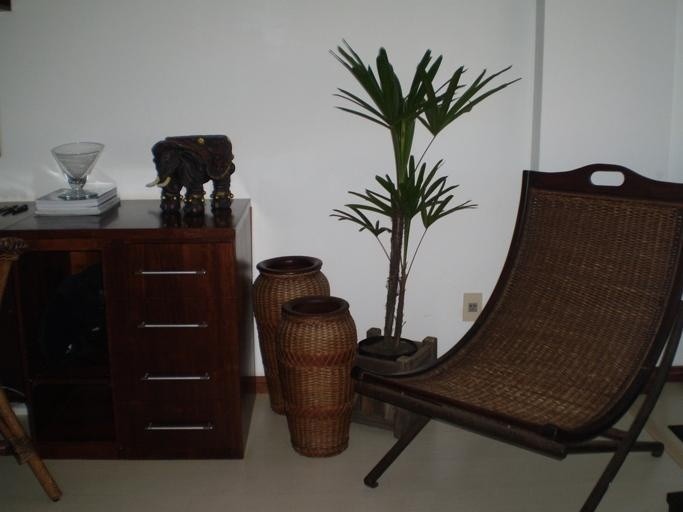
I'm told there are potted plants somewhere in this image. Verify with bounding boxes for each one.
[330,38,522,360]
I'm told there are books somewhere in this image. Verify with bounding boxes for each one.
[35,186,120,216]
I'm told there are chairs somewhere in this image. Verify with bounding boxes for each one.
[348,164,683,512]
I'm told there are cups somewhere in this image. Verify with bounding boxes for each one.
[51,142,105,200]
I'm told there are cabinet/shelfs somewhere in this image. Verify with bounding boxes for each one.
[1,198,258,459]
